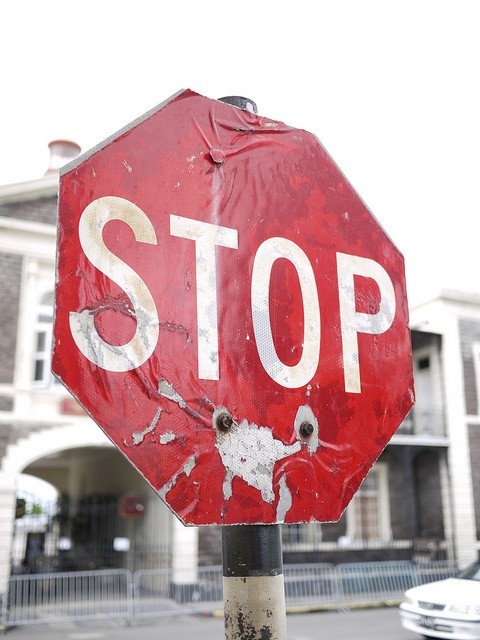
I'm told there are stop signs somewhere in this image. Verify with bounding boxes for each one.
[52,86,415,527]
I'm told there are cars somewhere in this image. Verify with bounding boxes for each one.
[398,557,480,640]
